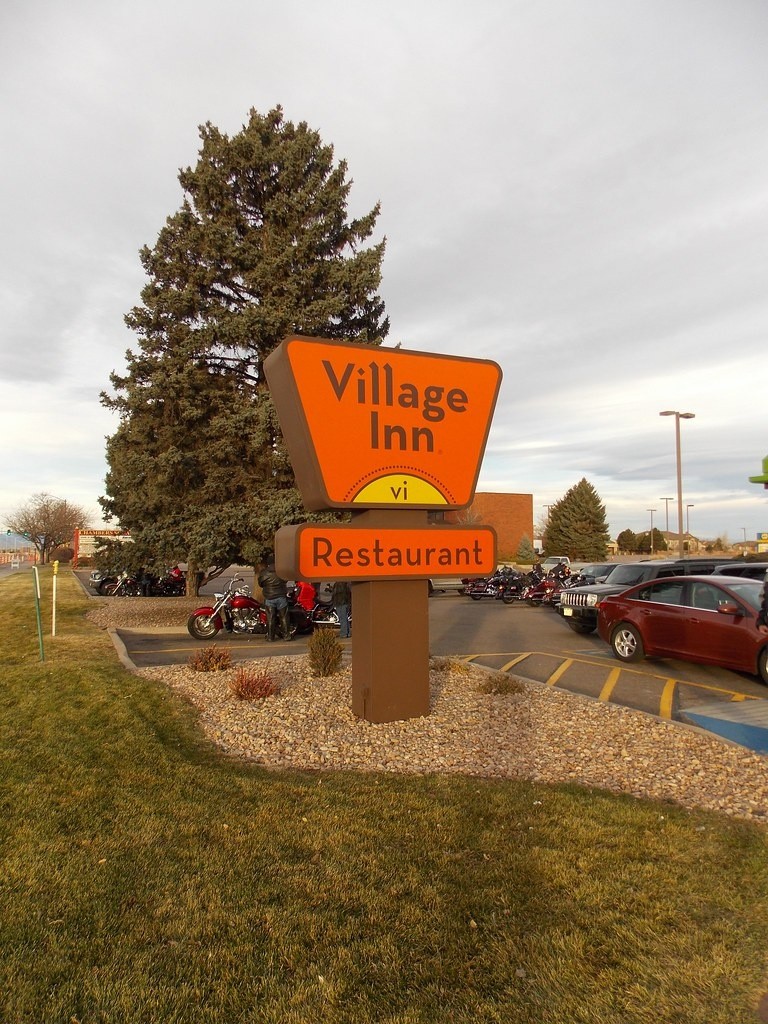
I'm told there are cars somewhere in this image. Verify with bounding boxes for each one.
[596,574,768,683]
[428,563,517,595]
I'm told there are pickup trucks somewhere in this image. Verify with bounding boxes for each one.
[89,558,204,595]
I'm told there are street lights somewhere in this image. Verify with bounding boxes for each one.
[687,505,694,556]
[660,498,673,556]
[647,509,657,556]
[740,528,747,551]
[660,410,695,558]
[543,505,555,524]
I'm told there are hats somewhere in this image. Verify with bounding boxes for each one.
[267,556,274,564]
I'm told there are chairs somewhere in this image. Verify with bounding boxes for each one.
[695,587,715,610]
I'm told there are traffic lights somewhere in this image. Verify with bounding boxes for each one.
[7,530,11,536]
[24,530,28,536]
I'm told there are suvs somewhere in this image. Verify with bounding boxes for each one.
[541,556,570,570]
[552,556,768,633]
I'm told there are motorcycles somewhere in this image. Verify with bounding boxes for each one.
[458,565,586,607]
[187,573,352,639]
[104,567,187,597]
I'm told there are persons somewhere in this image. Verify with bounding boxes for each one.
[553,562,571,578]
[533,558,542,573]
[253,547,272,604]
[258,555,293,641]
[331,581,352,638]
[171,560,181,581]
